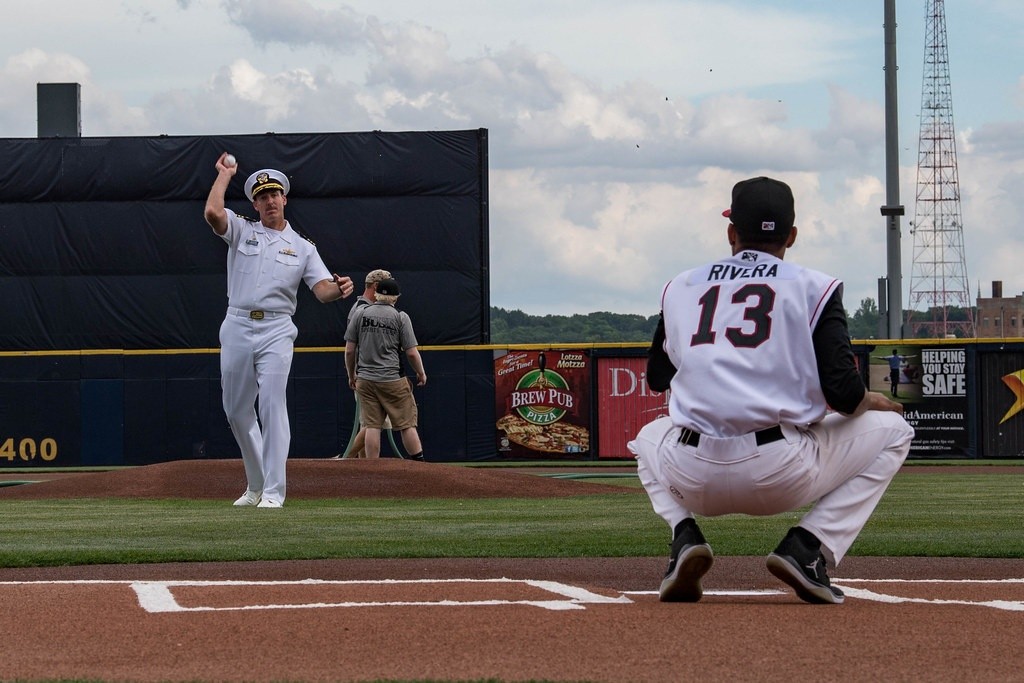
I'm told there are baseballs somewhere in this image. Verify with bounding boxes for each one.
[223,154,235,167]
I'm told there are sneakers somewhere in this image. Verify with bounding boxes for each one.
[258,498,284,508]
[766,531,845,603]
[658,525,713,602]
[234,485,265,507]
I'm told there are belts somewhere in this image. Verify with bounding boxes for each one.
[677,425,798,447]
[227,308,289,319]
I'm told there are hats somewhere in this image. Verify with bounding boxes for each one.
[377,278,399,295]
[365,270,394,283]
[243,169,291,203]
[723,177,795,232]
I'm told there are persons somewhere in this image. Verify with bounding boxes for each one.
[205,151,353,509]
[333,270,394,458]
[345,279,426,460]
[626,177,915,605]
[872,349,917,396]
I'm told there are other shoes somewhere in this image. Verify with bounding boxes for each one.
[408,450,426,462]
